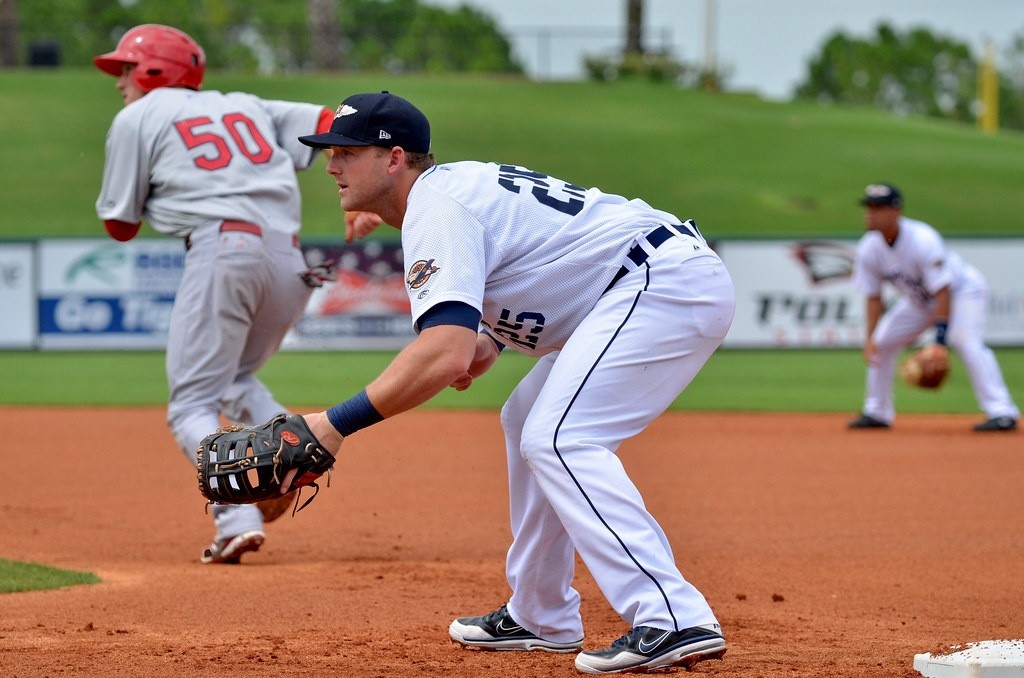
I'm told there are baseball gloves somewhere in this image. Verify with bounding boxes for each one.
[196,412,336,517]
[904,352,947,388]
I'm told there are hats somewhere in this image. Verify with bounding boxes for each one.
[857,180,904,208]
[297,90,432,154]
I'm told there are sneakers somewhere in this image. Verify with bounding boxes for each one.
[258,489,297,522]
[200,506,265,563]
[449,608,583,652]
[574,618,728,674]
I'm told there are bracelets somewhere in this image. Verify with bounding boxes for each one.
[479,328,506,352]
[326,390,387,437]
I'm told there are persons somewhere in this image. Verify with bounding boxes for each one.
[278,91,736,674]
[95,22,383,564]
[850,181,1018,431]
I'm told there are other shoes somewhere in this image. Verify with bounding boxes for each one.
[972,414,1016,431]
[847,414,889,428]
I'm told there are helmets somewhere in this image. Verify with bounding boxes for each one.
[92,24,208,92]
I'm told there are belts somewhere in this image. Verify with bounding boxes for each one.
[600,220,699,295]
[184,220,302,249]
